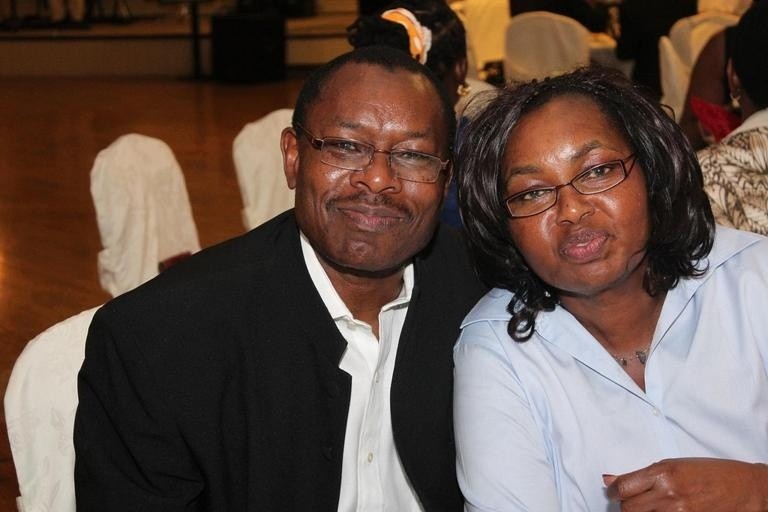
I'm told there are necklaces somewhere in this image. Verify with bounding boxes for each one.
[609,330,656,366]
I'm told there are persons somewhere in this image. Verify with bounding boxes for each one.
[614,1,699,100]
[447,64,767,511]
[694,4,767,235]
[72,48,483,511]
[344,0,471,225]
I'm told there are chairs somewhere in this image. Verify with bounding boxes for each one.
[5,304,102,511]
[91,132,201,301]
[232,107,301,234]
[445,0,744,135]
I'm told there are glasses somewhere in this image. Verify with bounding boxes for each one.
[298,128,448,184]
[502,153,642,219]
